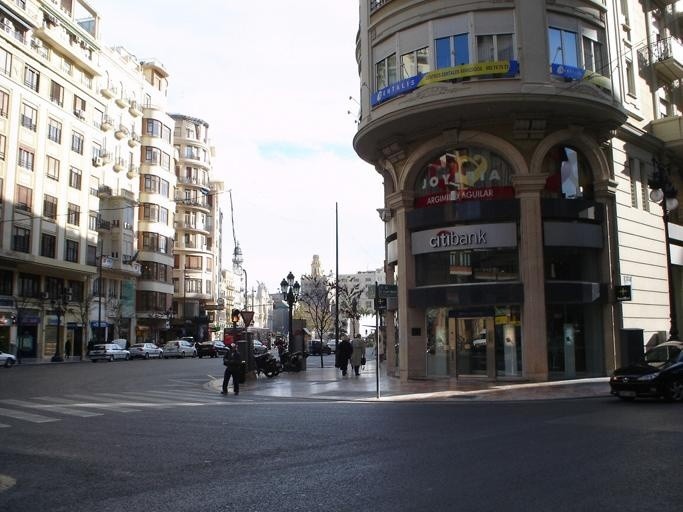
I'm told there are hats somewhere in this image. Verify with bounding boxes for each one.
[228,343,236,348]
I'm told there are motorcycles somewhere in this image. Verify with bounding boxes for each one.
[254,347,283,377]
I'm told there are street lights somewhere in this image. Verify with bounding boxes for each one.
[647,155,681,341]
[280,271,299,353]
[48,287,71,362]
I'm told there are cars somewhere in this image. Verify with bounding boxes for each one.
[326,339,342,353]
[88,343,130,362]
[472,328,487,349]
[127,342,163,360]
[162,340,197,359]
[196,340,230,358]
[606,339,683,404]
[252,339,268,351]
[306,339,331,355]
[0,350,16,368]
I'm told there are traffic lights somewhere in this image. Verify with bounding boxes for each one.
[231,308,240,322]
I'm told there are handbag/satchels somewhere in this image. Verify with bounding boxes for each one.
[335,362,340,367]
[361,355,366,365]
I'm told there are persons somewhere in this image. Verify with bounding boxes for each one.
[333,335,355,376]
[64,339,71,359]
[219,342,243,395]
[86,337,111,356]
[350,332,366,375]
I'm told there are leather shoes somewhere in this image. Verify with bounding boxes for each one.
[221,391,227,393]
[235,392,238,395]
[343,371,360,375]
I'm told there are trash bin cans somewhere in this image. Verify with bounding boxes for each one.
[10,343,15,355]
[240,363,245,383]
